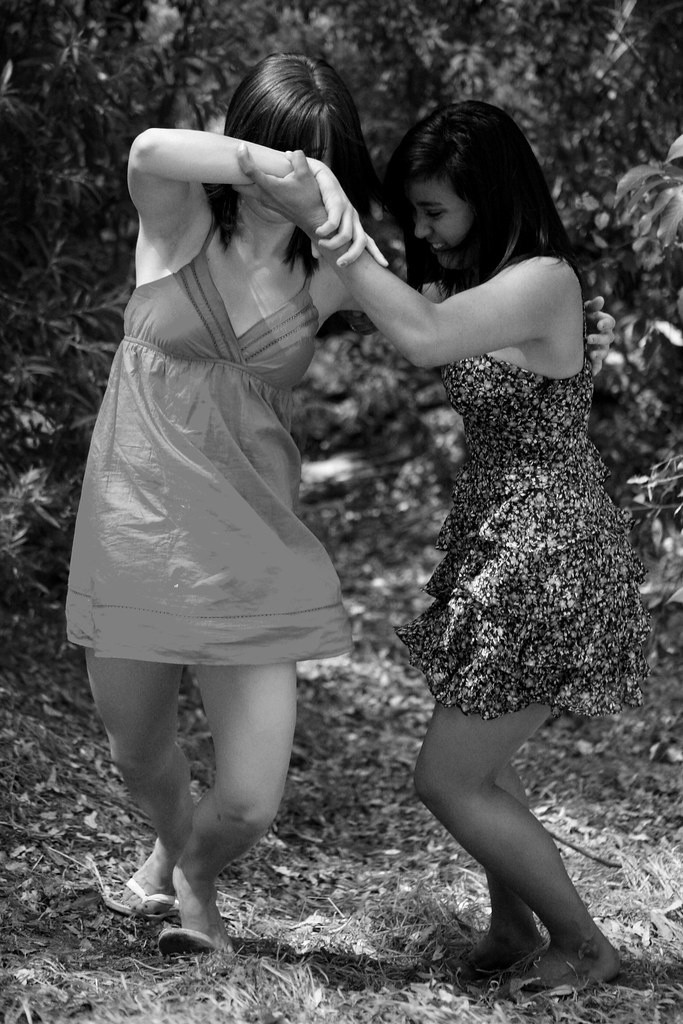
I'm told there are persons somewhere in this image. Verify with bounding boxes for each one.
[65,52,616,957]
[232,99,653,1003]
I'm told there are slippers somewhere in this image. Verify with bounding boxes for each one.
[105,876,180,920]
[439,946,517,985]
[155,927,217,956]
[493,957,586,1003]
